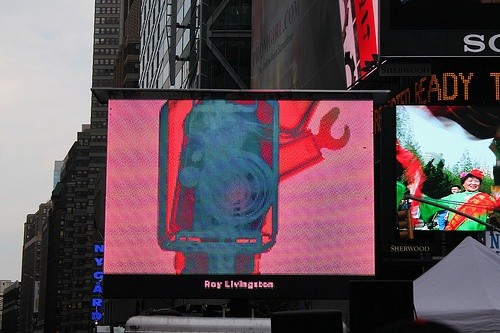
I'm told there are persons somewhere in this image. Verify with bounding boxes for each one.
[451,185,462,193]
[419,169,496,231]
